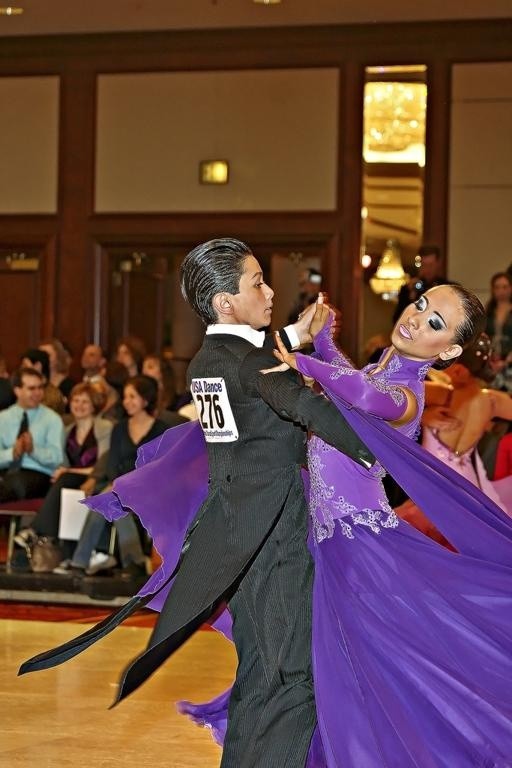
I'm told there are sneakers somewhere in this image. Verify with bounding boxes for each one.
[85,554,117,575]
[14,528,39,549]
[120,569,137,578]
[52,565,85,577]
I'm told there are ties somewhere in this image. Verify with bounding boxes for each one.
[4,413,28,481]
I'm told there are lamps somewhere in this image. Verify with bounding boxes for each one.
[199,159,229,186]
[363,65,427,152]
[369,239,410,303]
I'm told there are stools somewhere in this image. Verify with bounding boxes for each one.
[0,498,45,575]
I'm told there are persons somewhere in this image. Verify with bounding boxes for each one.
[18,236,376,767]
[392,245,450,327]
[286,268,323,325]
[483,272,511,392]
[1,338,178,575]
[78,283,512,767]
[391,332,512,554]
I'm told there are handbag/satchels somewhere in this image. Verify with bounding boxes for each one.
[27,534,71,573]
